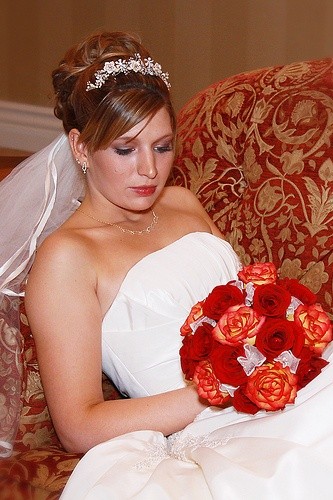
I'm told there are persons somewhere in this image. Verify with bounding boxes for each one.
[1,32,333,500]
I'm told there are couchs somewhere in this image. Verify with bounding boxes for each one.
[0,58,333,500]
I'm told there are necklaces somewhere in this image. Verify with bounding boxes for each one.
[76,206,160,235]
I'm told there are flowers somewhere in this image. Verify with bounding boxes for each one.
[179,261,333,413]
